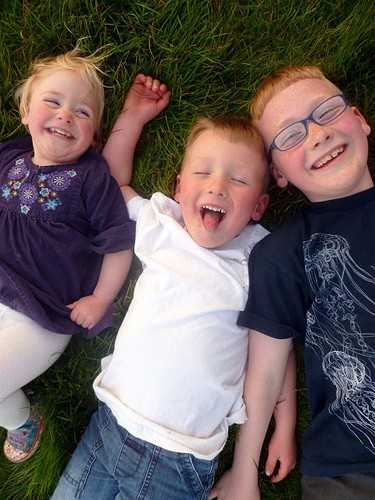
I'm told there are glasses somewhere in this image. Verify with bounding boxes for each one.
[267,94,348,164]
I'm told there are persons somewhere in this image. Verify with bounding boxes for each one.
[53,72,296,496]
[1,36,135,462]
[210,64,375,496]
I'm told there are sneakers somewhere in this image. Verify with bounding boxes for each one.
[3,405,49,463]
